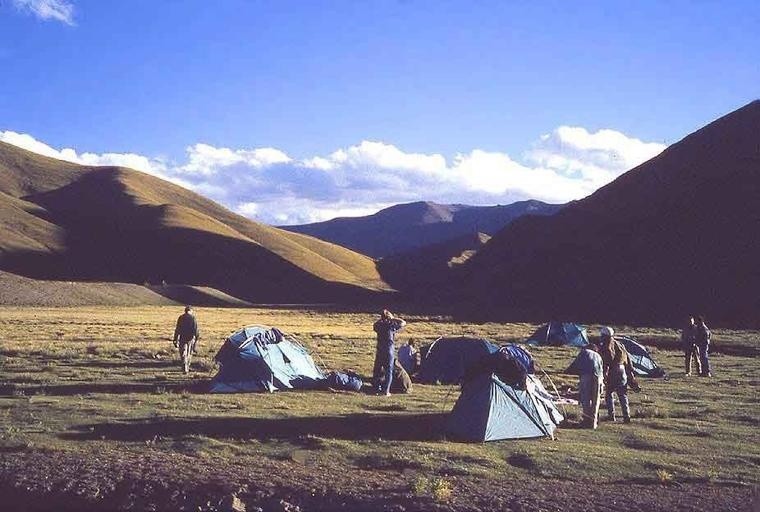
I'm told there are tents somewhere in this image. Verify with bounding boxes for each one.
[564,336,665,377]
[446,350,565,442]
[527,321,589,348]
[419,335,503,385]
[214,323,267,366]
[207,327,331,393]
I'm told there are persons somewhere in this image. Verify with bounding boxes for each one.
[697,315,713,377]
[564,341,603,429]
[371,309,407,396]
[173,304,200,375]
[395,338,420,377]
[679,315,702,376]
[598,326,640,422]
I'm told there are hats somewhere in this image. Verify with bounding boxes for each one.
[601,326,614,337]
